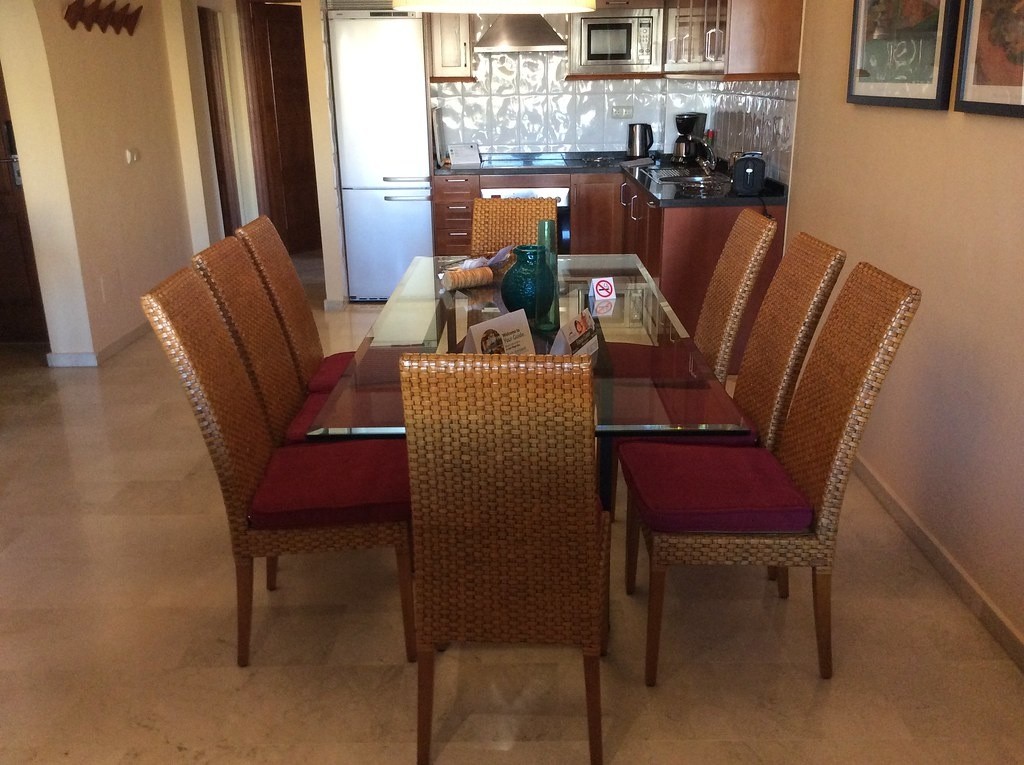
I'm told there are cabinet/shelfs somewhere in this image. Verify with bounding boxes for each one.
[433,175,480,255]
[430,13,471,78]
[662,0,803,82]
[570,173,624,255]
[620,174,787,375]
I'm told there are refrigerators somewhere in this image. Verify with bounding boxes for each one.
[327,9,433,301]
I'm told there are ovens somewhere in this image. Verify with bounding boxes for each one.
[481,188,571,255]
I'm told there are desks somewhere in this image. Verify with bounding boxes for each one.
[305,252,750,654]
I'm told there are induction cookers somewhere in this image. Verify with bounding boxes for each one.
[481,152,573,175]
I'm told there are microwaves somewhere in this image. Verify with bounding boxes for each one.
[582,18,652,65]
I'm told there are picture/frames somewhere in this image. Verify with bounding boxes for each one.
[846,0,961,111]
[954,0,1024,119]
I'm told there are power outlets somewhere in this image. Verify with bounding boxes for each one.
[612,106,623,119]
[623,106,633,119]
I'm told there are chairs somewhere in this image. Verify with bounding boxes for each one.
[236,215,439,394]
[193,236,405,572]
[619,261,921,689]
[397,352,613,765]
[594,209,778,386]
[139,262,416,668]
[466,197,561,334]
[598,230,846,581]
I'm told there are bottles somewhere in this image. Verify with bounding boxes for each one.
[501,245,554,319]
[535,220,560,332]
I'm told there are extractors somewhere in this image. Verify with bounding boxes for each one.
[474,14,568,53]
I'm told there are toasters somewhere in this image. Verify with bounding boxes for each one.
[734,152,766,195]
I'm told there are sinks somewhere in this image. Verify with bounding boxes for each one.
[658,174,730,182]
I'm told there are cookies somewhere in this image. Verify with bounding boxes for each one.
[442,267,494,291]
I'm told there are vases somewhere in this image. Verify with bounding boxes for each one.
[501,244,553,320]
[535,220,560,337]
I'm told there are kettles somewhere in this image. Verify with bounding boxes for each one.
[627,123,653,160]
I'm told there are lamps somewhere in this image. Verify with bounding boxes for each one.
[391,0,596,14]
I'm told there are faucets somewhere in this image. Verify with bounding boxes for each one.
[686,134,717,176]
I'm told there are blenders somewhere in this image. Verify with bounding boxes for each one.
[673,115,699,167]
